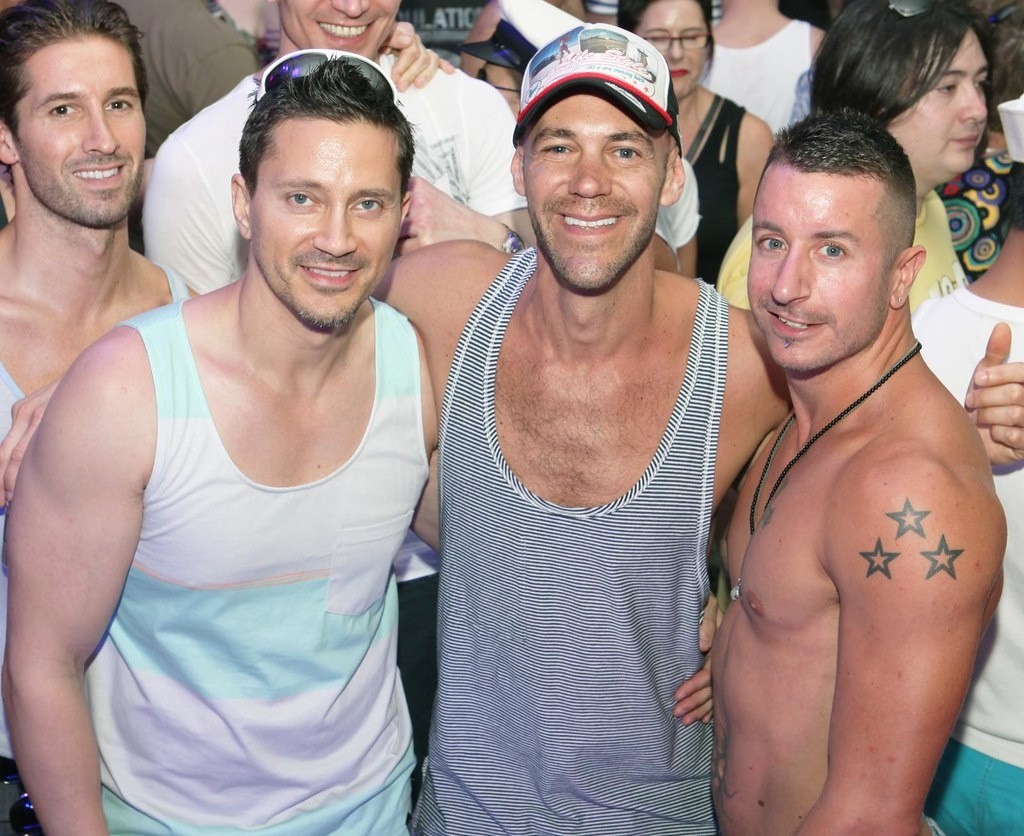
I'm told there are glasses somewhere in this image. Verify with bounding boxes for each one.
[879,0,970,21]
[255,49,398,107]
[477,69,518,93]
[644,33,710,51]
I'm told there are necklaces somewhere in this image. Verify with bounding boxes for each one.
[730,339,922,602]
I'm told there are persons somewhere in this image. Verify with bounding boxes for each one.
[718,0,996,313]
[912,96,1024,836]
[707,109,1008,836]
[700,0,828,139]
[0,0,455,255]
[382,0,523,119]
[0,57,724,835]
[142,0,533,298]
[615,0,772,280]
[0,19,1024,836]
[0,0,200,836]
[460,0,700,279]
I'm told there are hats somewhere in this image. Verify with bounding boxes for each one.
[513,23,682,157]
[996,93,1024,165]
[457,0,589,76]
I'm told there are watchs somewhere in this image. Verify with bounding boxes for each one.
[502,223,526,254]
